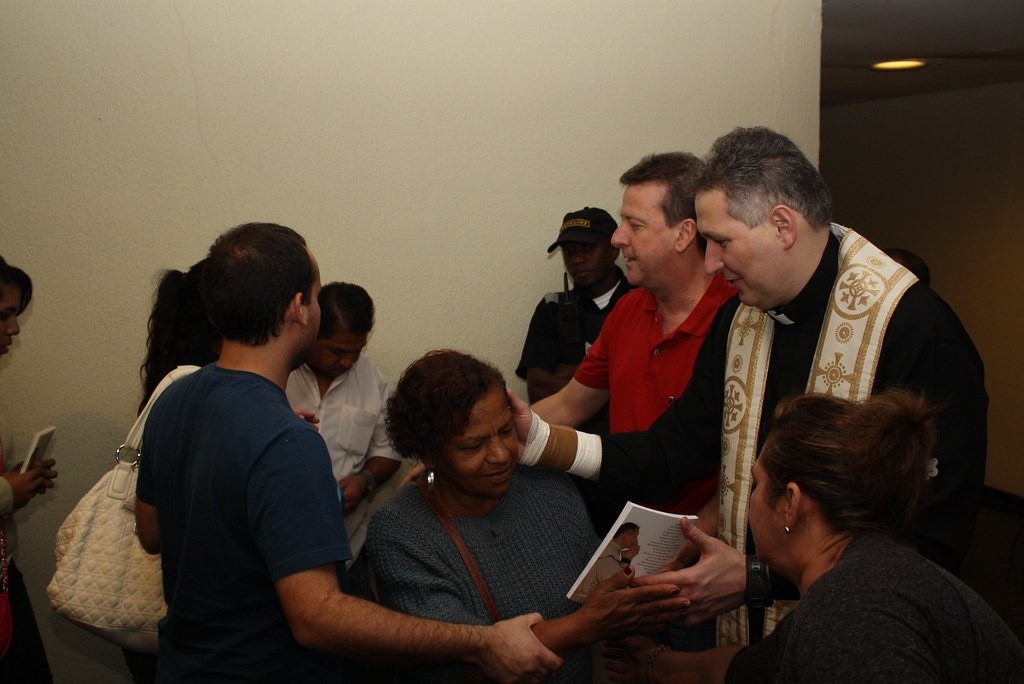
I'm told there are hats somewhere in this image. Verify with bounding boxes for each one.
[547,206,618,253]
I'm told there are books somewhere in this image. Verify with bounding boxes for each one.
[20,426,56,475]
[566,500,698,606]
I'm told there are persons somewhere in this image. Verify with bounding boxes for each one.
[135,223,562,684]
[0,256,57,684]
[571,522,640,605]
[506,124,988,645]
[605,389,1024,684]
[350,347,691,684]
[400,151,738,540]
[883,248,930,289]
[516,206,635,409]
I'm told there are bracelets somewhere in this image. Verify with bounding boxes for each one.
[646,644,668,681]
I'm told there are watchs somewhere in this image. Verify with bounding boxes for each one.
[745,557,773,609]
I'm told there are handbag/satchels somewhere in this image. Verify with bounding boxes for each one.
[0,590,12,657]
[44,365,202,656]
